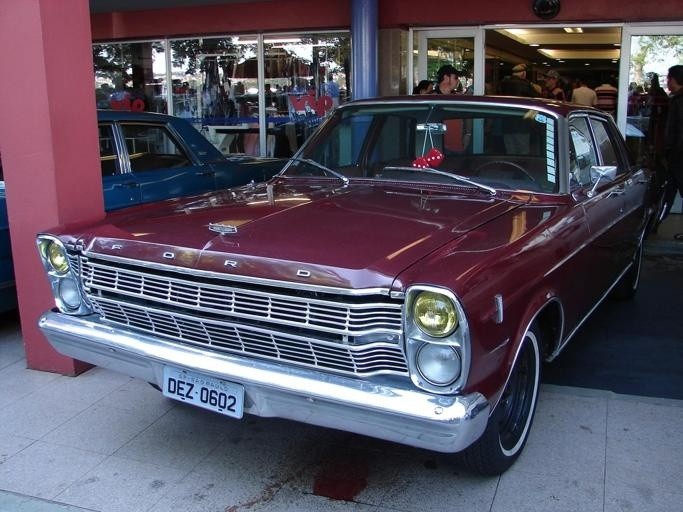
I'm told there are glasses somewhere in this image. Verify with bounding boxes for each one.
[544,77,552,80]
[449,75,458,80]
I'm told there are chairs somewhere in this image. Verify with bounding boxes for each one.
[471,154,546,181]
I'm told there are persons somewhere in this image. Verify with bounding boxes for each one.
[94,70,341,121]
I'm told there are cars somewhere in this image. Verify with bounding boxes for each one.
[0,107,288,321]
[33,93,666,471]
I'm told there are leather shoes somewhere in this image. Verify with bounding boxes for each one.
[648,228,658,234]
[675,233,683,241]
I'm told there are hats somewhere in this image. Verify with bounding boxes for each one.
[437,65,463,75]
[512,64,528,72]
[543,70,560,79]
